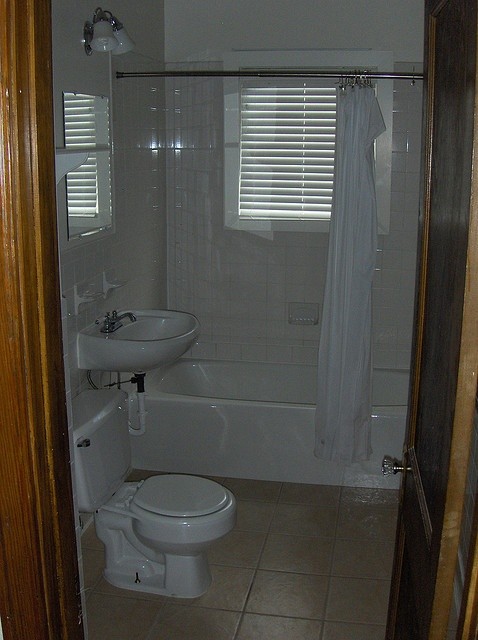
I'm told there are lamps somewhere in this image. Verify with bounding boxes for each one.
[83,6,136,56]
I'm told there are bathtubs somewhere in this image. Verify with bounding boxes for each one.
[130,357,409,491]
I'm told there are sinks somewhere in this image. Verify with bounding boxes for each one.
[77,309,199,370]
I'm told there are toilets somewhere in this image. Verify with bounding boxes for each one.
[72,389,237,599]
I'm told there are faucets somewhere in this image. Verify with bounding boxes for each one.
[104,310,136,331]
